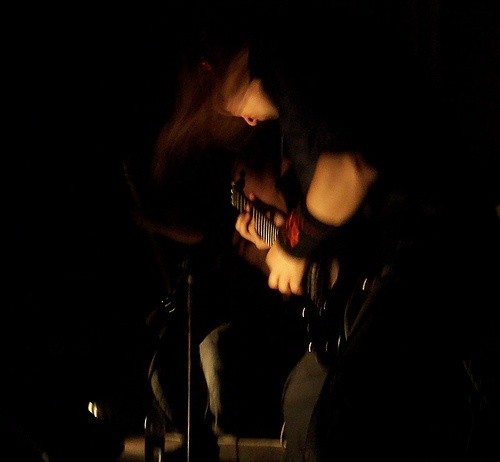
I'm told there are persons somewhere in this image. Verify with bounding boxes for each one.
[133,158,288,441]
[196,15,499,462]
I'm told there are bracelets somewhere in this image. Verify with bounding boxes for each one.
[276,199,337,258]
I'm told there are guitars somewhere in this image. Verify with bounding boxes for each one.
[231,157,341,293]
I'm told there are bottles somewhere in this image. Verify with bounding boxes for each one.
[145,399,165,462]
